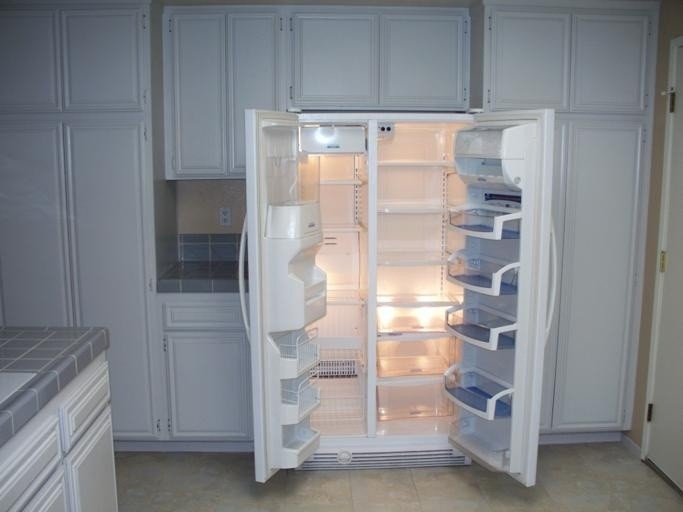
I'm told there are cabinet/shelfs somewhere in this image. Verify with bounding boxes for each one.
[160,294,258,454]
[165,3,287,180]
[2,2,179,451]
[288,6,470,111]
[1,353,120,511]
[473,0,666,450]
